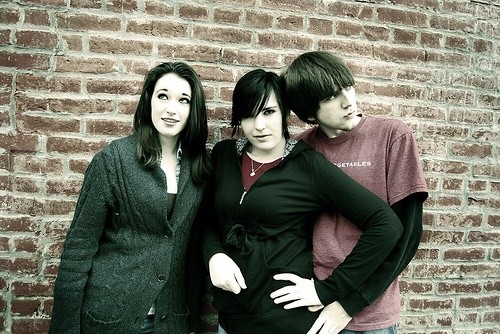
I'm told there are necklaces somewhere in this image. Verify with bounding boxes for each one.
[249,149,273,176]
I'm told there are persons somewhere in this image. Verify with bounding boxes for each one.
[279,52,429,334]
[198,68,404,334]
[46,62,220,334]
